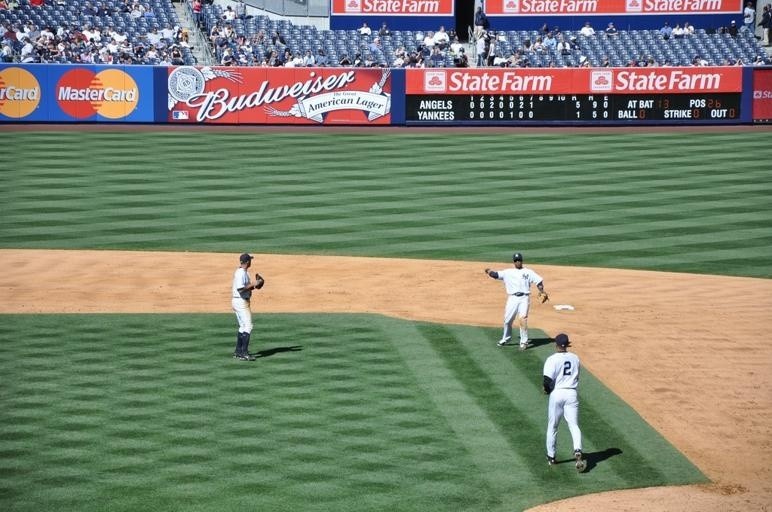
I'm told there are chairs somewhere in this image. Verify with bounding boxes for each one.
[1,1,317,66]
[315,30,771,67]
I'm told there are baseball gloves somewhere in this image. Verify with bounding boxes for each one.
[255,273,264,289]
[538,291,548,304]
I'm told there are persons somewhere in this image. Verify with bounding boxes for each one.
[484,252,548,352]
[229,253,265,361]
[538,333,585,473]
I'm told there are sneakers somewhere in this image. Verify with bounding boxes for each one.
[233,352,256,361]
[497,339,528,351]
[574,450,587,473]
[546,455,556,465]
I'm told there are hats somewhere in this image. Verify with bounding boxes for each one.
[556,334,568,347]
[512,253,522,260]
[240,254,253,264]
